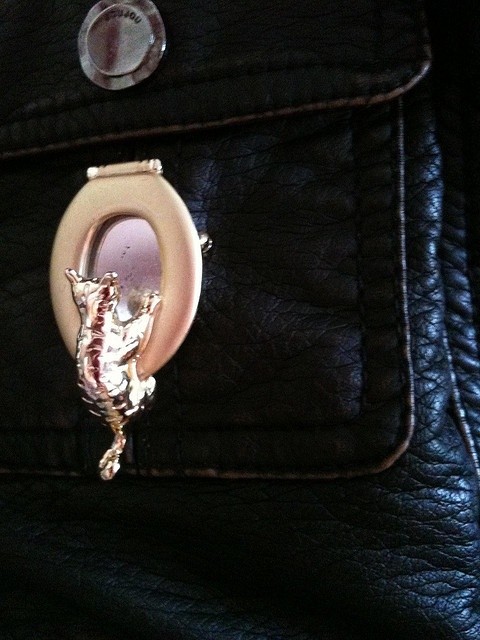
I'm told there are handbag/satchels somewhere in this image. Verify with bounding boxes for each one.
[1,0,480,639]
